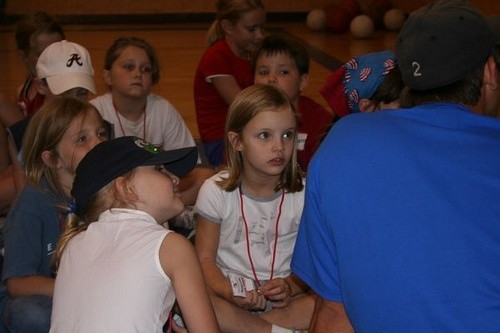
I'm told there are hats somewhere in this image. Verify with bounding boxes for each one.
[396,0,500,92]
[70,133,201,199]
[36,39,96,96]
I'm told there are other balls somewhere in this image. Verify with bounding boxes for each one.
[328,8,351,34]
[384,9,405,30]
[339,0,360,18]
[307,9,327,31]
[350,14,374,40]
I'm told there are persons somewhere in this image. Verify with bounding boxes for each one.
[0,97,114,332]
[330,46,409,119]
[0,39,114,215]
[241,32,337,176]
[169,84,332,333]
[286,2,499,332]
[87,36,215,214]
[0,13,67,125]
[194,0,278,172]
[49,131,223,333]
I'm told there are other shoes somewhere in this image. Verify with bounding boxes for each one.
[183,205,199,229]
